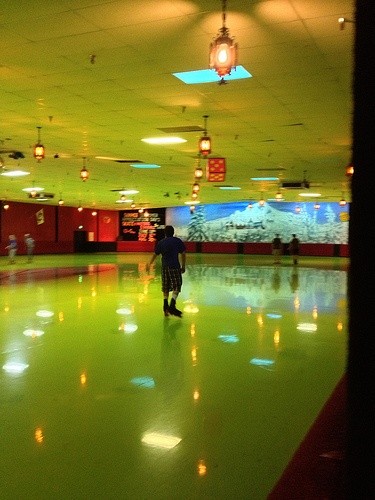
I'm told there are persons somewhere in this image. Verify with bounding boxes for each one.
[150,225,186,316]
[4,235,17,266]
[288,234,300,267]
[270,233,284,265]
[24,233,35,263]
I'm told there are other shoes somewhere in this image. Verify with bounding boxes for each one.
[163,304,168,316]
[168,306,182,319]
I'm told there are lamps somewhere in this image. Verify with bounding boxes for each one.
[81,157,88,182]
[33,126,45,161]
[190,115,211,213]
[209,0,236,86]
